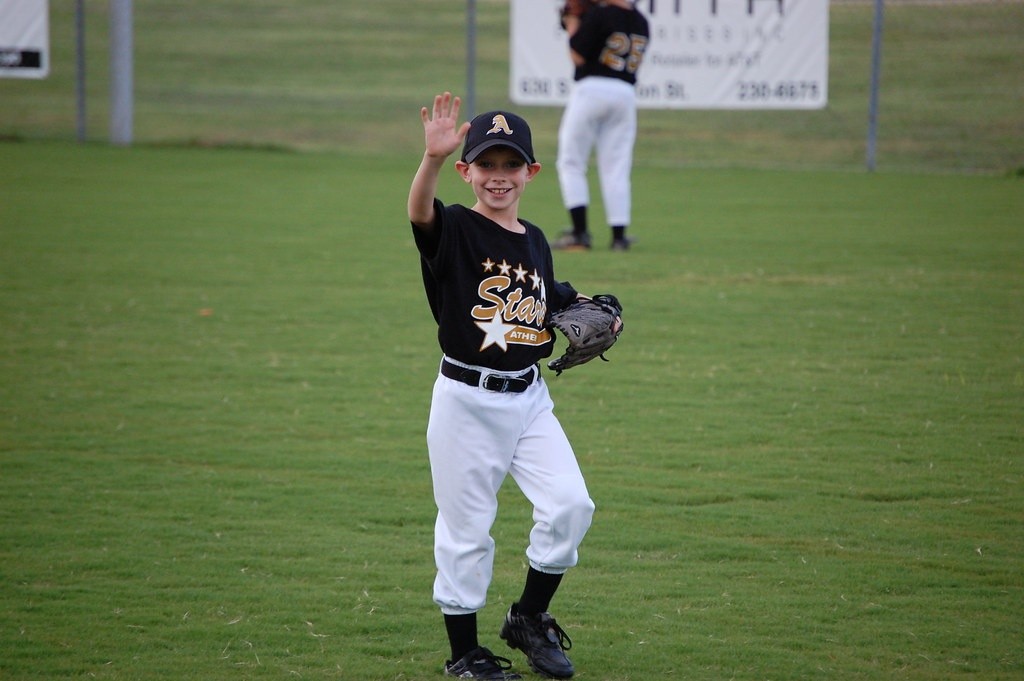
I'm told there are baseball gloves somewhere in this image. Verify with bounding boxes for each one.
[544,294,624,376]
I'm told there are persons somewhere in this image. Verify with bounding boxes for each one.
[549,0,650,249]
[407,90,621,681]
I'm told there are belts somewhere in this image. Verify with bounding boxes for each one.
[440,360,541,393]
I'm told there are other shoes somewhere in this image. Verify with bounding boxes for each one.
[554,234,592,251]
[612,240,628,249]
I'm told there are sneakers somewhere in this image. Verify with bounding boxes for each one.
[445,646,522,681]
[500,603,574,679]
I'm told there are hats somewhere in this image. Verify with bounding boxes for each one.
[459,111,535,163]
